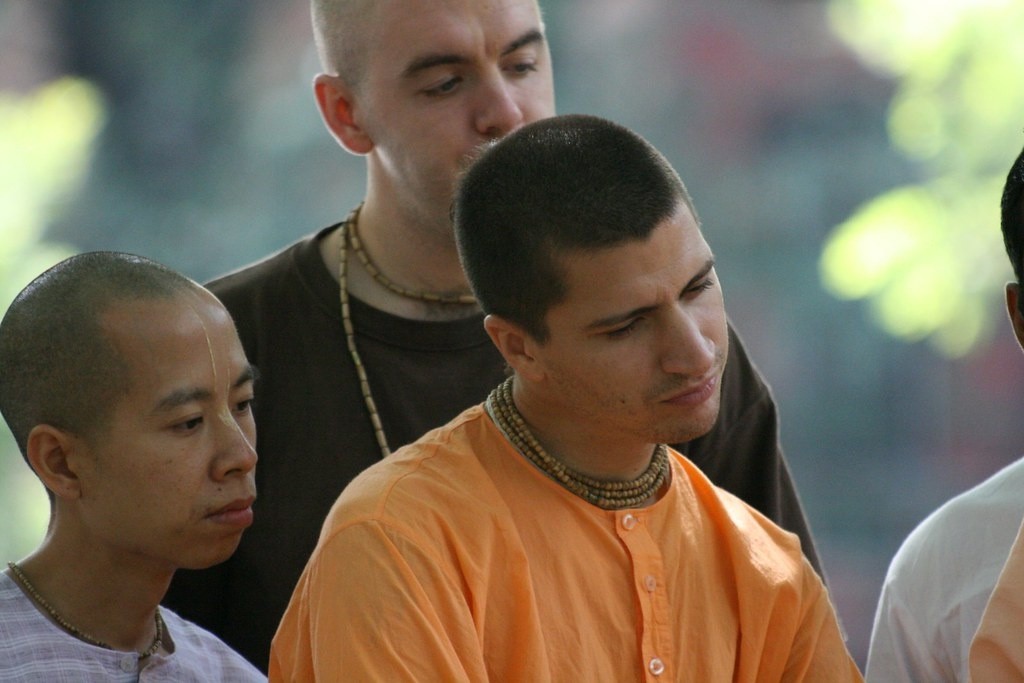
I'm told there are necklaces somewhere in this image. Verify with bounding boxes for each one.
[6,562,163,661]
[339,201,481,460]
[489,376,670,508]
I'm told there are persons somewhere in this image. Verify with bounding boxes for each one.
[163,0,826,675]
[272,115,864,682]
[0,252,268,683]
[865,149,1024,681]
